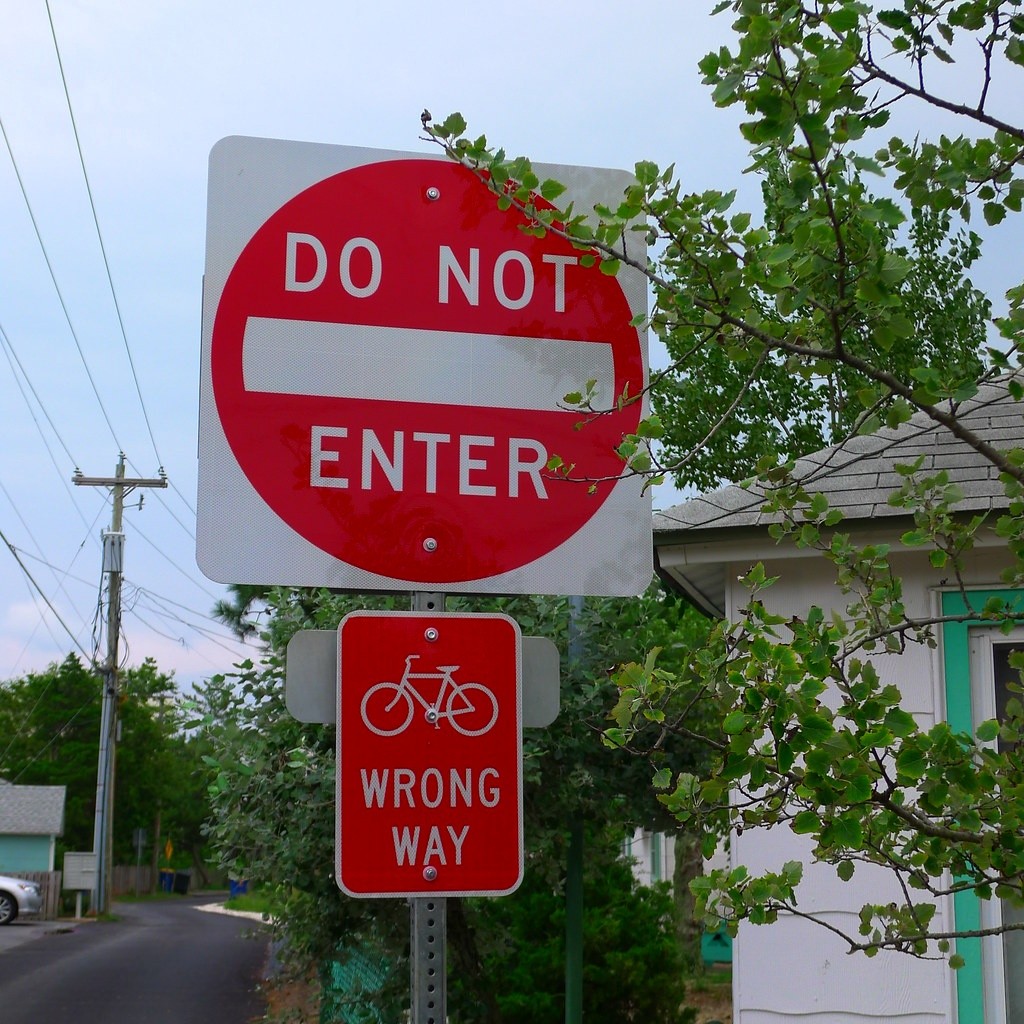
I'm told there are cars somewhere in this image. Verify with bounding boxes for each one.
[0,875,40,925]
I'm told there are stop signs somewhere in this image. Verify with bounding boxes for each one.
[196,134,655,598]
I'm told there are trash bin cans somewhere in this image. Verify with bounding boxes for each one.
[174,872,192,894]
[229,878,250,899]
[160,868,174,891]
